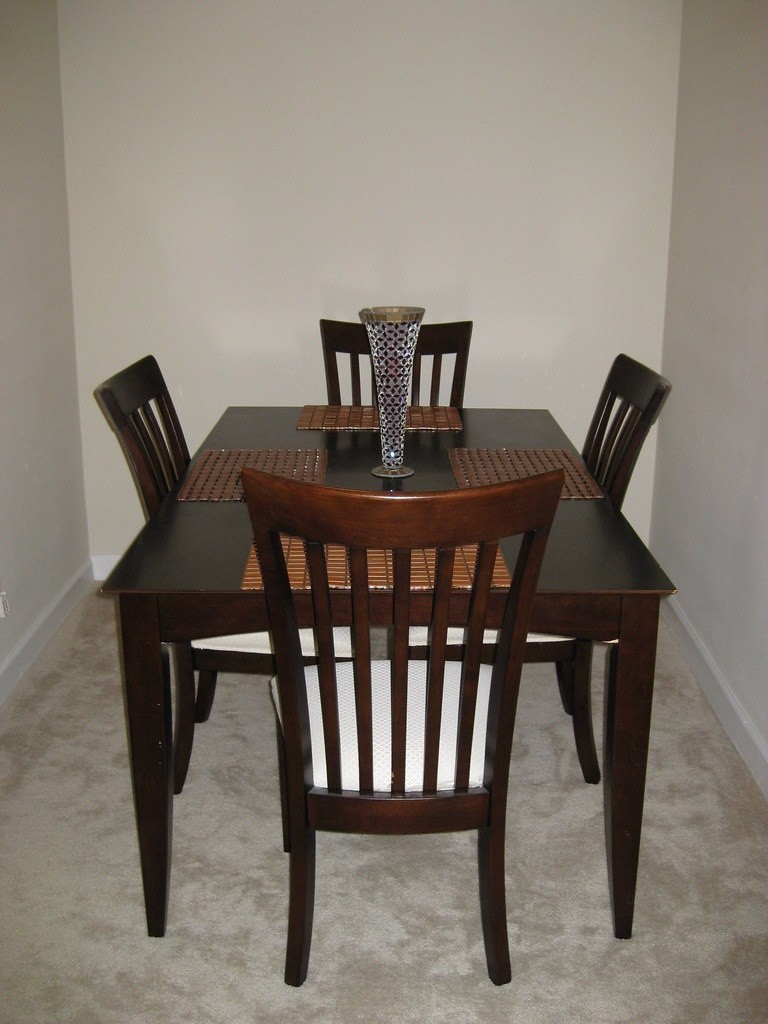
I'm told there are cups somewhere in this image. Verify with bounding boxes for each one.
[359,307,424,478]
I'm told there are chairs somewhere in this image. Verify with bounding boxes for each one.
[240,467,565,987]
[406,353,672,784]
[319,319,473,408]
[95,355,360,796]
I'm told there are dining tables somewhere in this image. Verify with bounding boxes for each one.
[98,406,677,940]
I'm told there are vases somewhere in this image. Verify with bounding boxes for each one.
[358,307,426,477]
